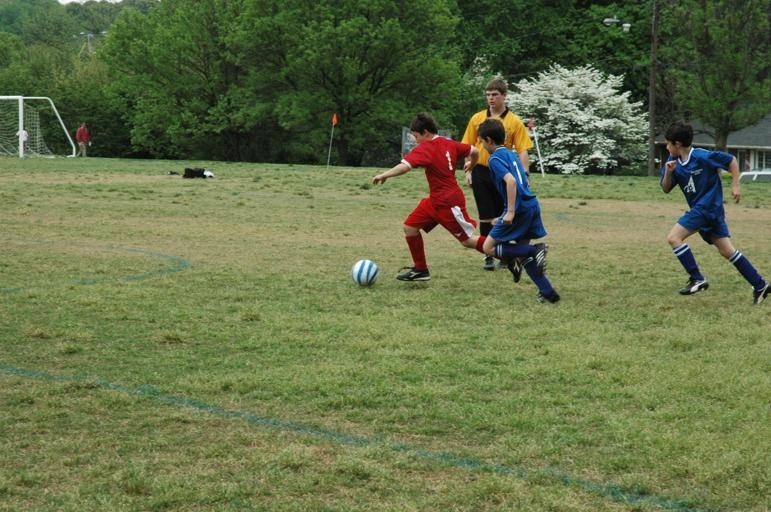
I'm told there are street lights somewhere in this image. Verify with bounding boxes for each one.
[601,17,658,178]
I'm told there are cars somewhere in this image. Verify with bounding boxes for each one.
[737,170,771,182]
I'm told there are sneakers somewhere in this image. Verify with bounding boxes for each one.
[483,242,549,283]
[753,282,770,305]
[537,294,560,303]
[395,269,431,281]
[679,277,708,295]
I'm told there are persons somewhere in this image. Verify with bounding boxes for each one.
[460,78,536,271]
[475,119,561,304]
[15,130,28,154]
[658,116,771,305]
[75,121,92,157]
[369,111,526,285]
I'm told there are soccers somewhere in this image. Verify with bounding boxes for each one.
[352,260,379,285]
